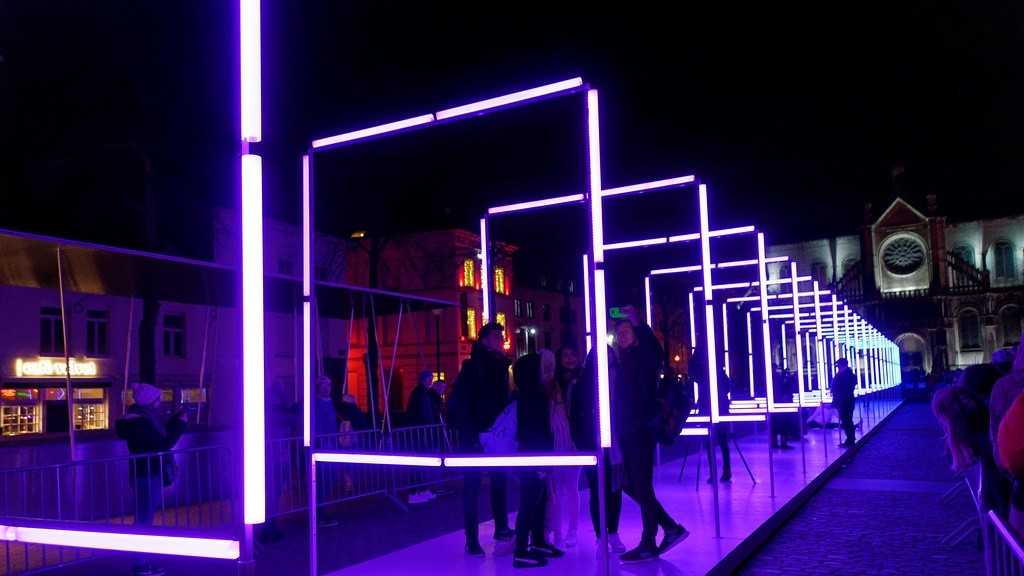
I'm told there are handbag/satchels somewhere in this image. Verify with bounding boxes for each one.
[163,454,177,486]
[639,357,692,446]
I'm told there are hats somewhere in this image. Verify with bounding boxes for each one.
[315,377,331,384]
[836,358,848,367]
[131,383,161,406]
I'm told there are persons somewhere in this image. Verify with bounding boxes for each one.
[402,370,437,504]
[335,394,371,497]
[931,328,1024,549]
[571,303,691,565]
[829,358,856,447]
[547,343,580,547]
[511,348,565,569]
[706,353,733,484]
[445,322,515,558]
[114,382,188,576]
[767,359,819,450]
[427,378,454,495]
[298,375,337,527]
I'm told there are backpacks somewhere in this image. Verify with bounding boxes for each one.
[445,358,485,428]
[479,401,519,454]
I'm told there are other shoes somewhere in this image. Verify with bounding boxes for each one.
[554,534,563,546]
[408,493,428,503]
[608,533,626,552]
[565,532,578,546]
[839,442,855,447]
[132,565,166,576]
[318,519,338,527]
[596,537,607,559]
[419,491,436,499]
[771,445,781,448]
[720,472,732,482]
[781,445,793,450]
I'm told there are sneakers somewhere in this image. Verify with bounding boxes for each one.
[619,545,659,563]
[513,556,548,569]
[465,540,485,556]
[658,524,690,555]
[493,529,516,540]
[530,544,564,557]
